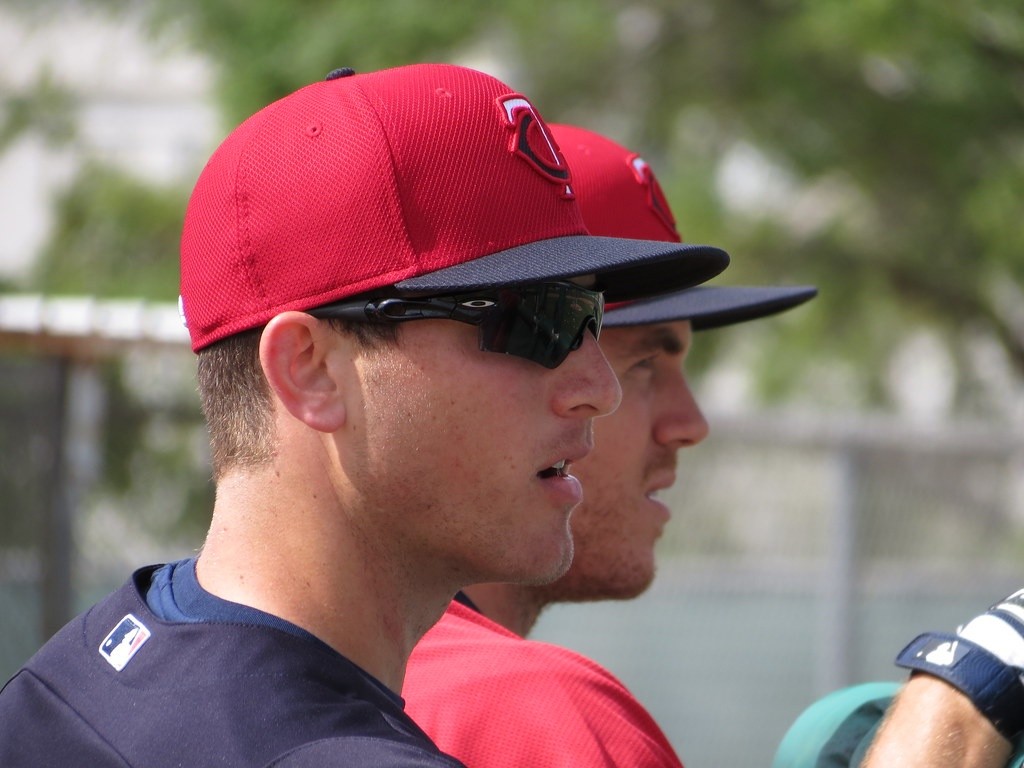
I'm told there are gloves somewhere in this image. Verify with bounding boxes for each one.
[893,587,1024,749]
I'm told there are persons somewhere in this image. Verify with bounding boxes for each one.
[400,125,820,768]
[1,64,1024,768]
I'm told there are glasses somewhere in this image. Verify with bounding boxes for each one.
[304,277,605,369]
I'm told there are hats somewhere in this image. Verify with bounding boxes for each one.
[544,123,819,331]
[180,64,731,354]
